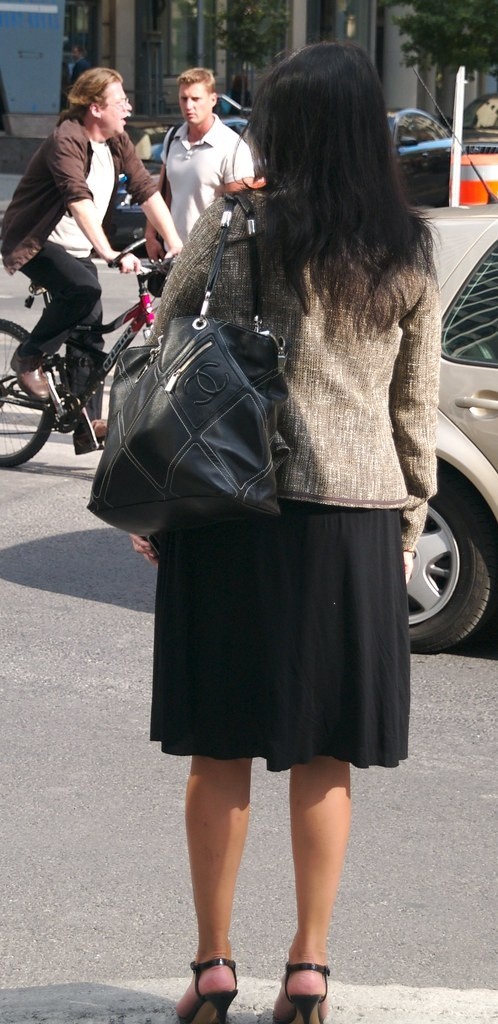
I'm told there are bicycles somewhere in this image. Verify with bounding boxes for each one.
[0,235,174,466]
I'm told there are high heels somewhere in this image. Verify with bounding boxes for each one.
[177,959,239,1024]
[272,962,331,1024]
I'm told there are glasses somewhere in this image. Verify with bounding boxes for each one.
[99,98,130,107]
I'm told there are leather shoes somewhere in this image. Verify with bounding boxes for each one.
[10,345,50,401]
[73,419,107,455]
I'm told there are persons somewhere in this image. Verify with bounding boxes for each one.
[71,45,91,84]
[145,67,254,261]
[228,74,252,117]
[129,43,442,1023]
[0,67,184,454]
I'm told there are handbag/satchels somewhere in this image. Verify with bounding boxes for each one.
[86,194,289,540]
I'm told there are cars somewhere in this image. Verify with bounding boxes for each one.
[409,203,498,654]
[461,95,498,140]
[387,106,454,210]
[104,93,252,256]
